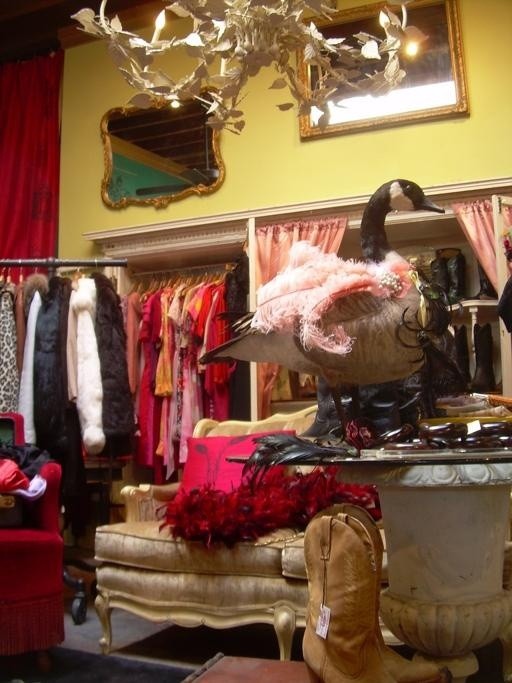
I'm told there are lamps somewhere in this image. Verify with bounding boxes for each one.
[66,0,427,134]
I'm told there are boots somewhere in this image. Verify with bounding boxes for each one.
[432,253,497,303]
[302,503,440,681]
[450,324,497,392]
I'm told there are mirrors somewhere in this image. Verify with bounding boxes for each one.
[100,88,225,211]
[289,1,473,142]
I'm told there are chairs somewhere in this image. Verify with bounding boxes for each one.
[0,411,66,683]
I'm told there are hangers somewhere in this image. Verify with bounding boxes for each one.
[0,260,240,293]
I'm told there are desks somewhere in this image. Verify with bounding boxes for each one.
[223,442,512,683]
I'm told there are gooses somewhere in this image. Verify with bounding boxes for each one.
[200,179,445,446]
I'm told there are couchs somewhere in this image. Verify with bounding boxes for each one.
[96,404,408,661]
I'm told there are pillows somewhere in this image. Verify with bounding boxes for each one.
[178,433,292,503]
